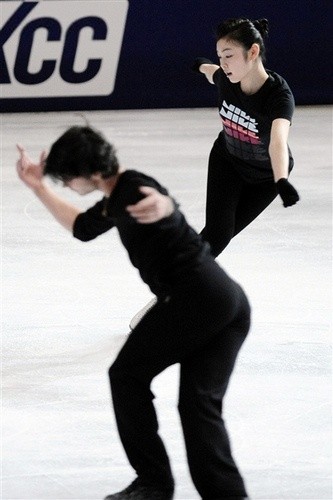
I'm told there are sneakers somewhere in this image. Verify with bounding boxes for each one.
[103,478,174,499]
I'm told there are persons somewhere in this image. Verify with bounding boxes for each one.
[13,124,250,499]
[175,19,300,260]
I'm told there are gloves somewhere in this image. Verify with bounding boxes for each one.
[191,56,212,75]
[277,178,299,208]
